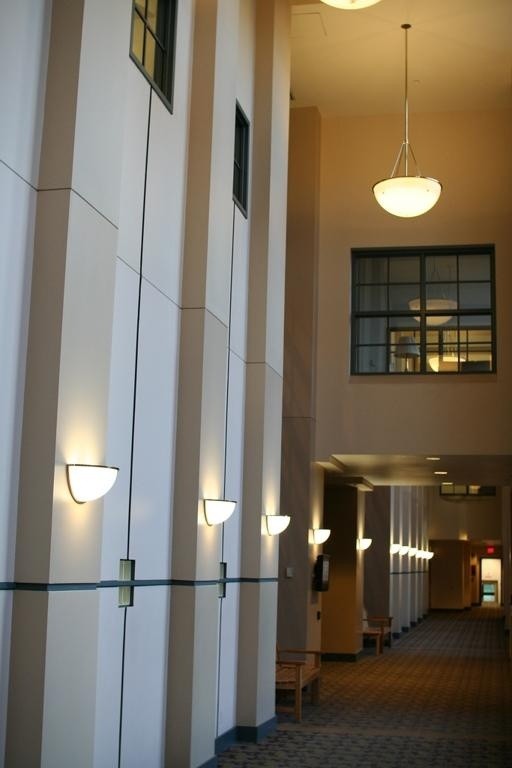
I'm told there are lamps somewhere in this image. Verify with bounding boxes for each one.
[393,336,420,372]
[370,22,443,220]
[407,255,458,328]
[65,457,333,550]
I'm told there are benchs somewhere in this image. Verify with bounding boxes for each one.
[274,640,325,720]
[361,606,394,655]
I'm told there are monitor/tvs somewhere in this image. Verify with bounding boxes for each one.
[312,553,331,591]
